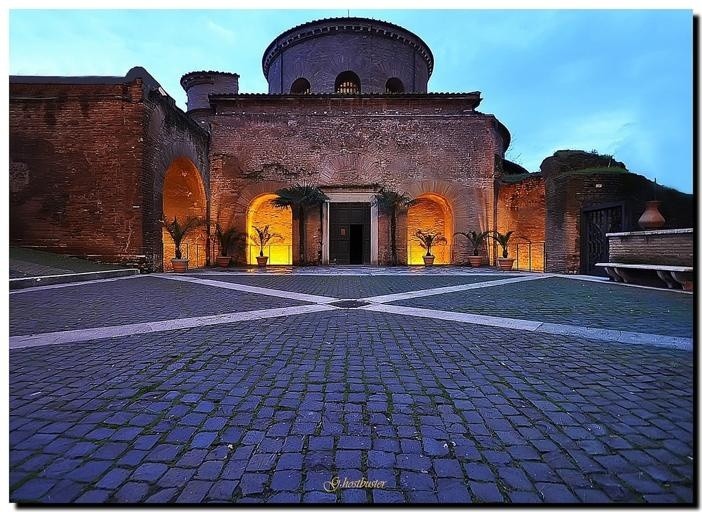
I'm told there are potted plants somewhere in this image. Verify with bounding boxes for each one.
[453,229,516,271]
[415,229,448,267]
[157,213,272,273]
